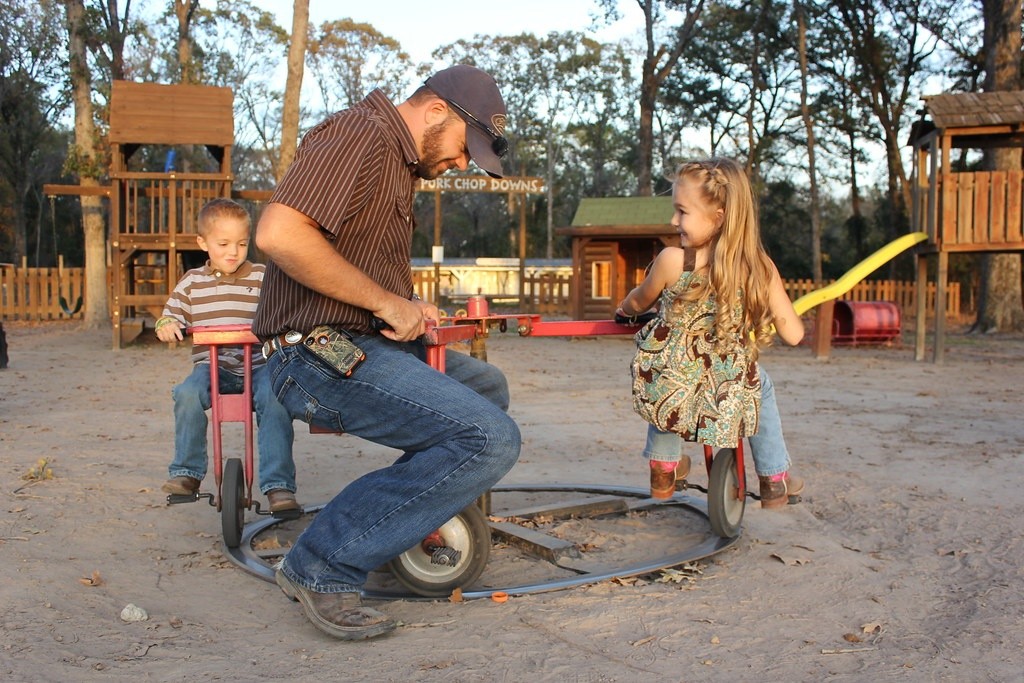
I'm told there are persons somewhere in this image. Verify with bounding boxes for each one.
[153,198,301,513]
[617,158,806,512]
[247,64,523,640]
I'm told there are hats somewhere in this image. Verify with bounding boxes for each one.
[425,62,508,181]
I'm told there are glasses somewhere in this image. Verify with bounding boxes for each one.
[447,98,510,159]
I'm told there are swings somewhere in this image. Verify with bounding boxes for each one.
[48,195,84,315]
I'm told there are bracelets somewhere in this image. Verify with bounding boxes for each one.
[155,316,176,331]
[619,298,635,318]
[412,293,421,300]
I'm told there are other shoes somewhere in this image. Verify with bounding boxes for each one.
[760,475,805,512]
[159,475,200,496]
[650,454,692,500]
[274,567,396,641]
[267,488,300,513]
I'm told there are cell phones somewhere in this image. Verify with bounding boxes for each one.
[303,324,365,376]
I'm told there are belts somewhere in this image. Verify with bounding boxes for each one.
[261,330,308,361]
